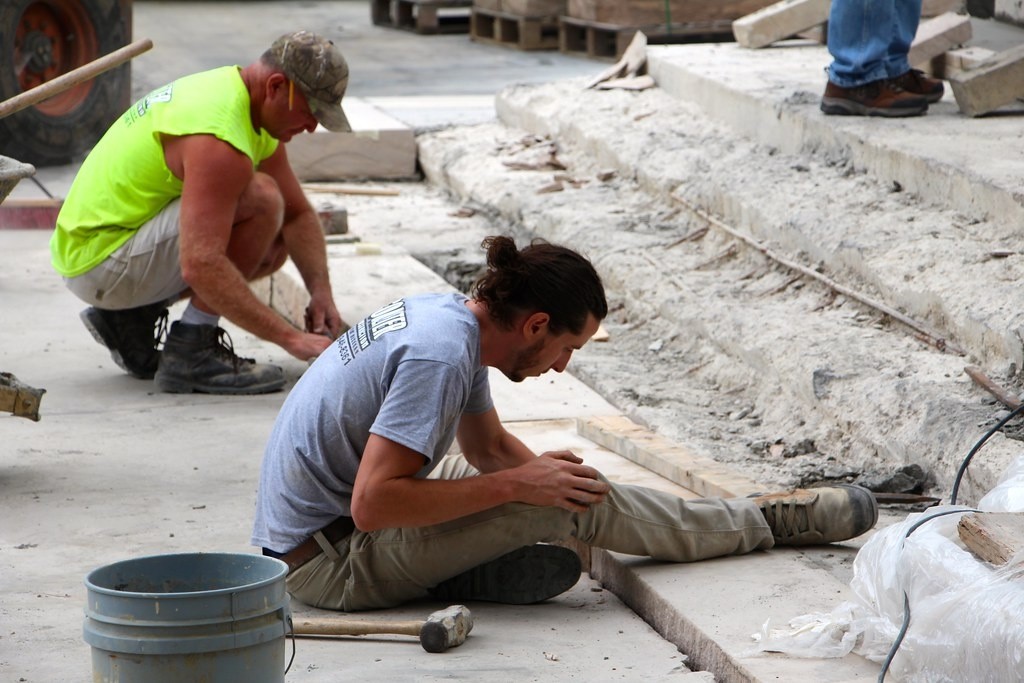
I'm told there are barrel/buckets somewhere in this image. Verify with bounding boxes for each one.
[83,551,296,683]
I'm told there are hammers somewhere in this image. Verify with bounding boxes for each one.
[288,605,475,654]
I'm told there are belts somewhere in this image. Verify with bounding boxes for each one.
[278,516,351,573]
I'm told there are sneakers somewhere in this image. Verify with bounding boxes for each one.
[81,301,163,379]
[160,320,287,395]
[752,485,880,548]
[440,545,584,604]
[821,68,946,117]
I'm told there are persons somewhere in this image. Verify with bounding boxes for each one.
[820,0,944,117]
[251,237,879,612]
[50,32,356,394]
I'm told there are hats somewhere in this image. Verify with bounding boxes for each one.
[270,31,353,134]
